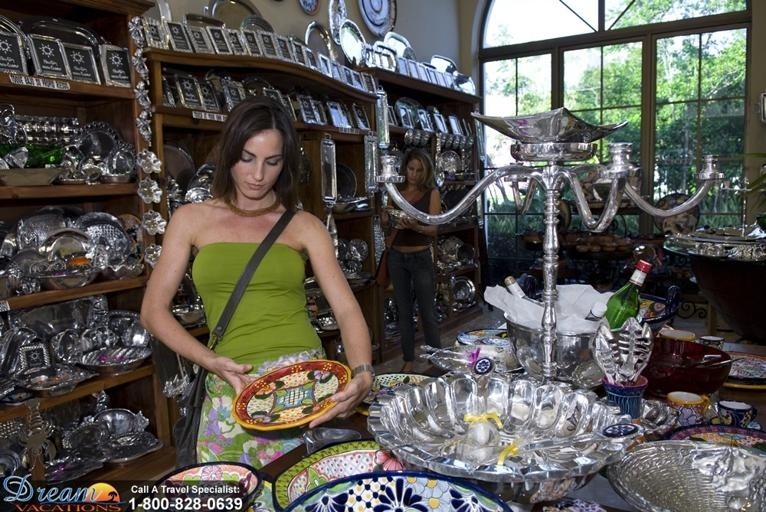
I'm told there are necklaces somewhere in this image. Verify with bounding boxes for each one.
[224,191,282,218]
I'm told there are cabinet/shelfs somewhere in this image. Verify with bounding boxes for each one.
[0,0,480,512]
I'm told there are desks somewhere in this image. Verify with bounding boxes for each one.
[257,366,449,487]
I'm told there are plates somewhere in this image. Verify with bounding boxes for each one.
[305,151,476,361]
[0,120,165,486]
[123,328,766,512]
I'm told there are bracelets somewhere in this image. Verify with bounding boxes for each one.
[350,365,376,381]
[416,225,424,234]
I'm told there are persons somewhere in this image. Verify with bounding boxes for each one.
[380,151,442,373]
[139,96,374,469]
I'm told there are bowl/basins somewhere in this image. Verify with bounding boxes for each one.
[430,292,766,398]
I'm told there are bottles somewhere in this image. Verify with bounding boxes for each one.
[504,276,546,309]
[0,114,82,169]
[605,260,652,330]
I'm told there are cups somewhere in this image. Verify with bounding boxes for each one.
[601,374,755,428]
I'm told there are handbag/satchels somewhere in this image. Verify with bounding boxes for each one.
[172,365,209,471]
[375,250,391,288]
